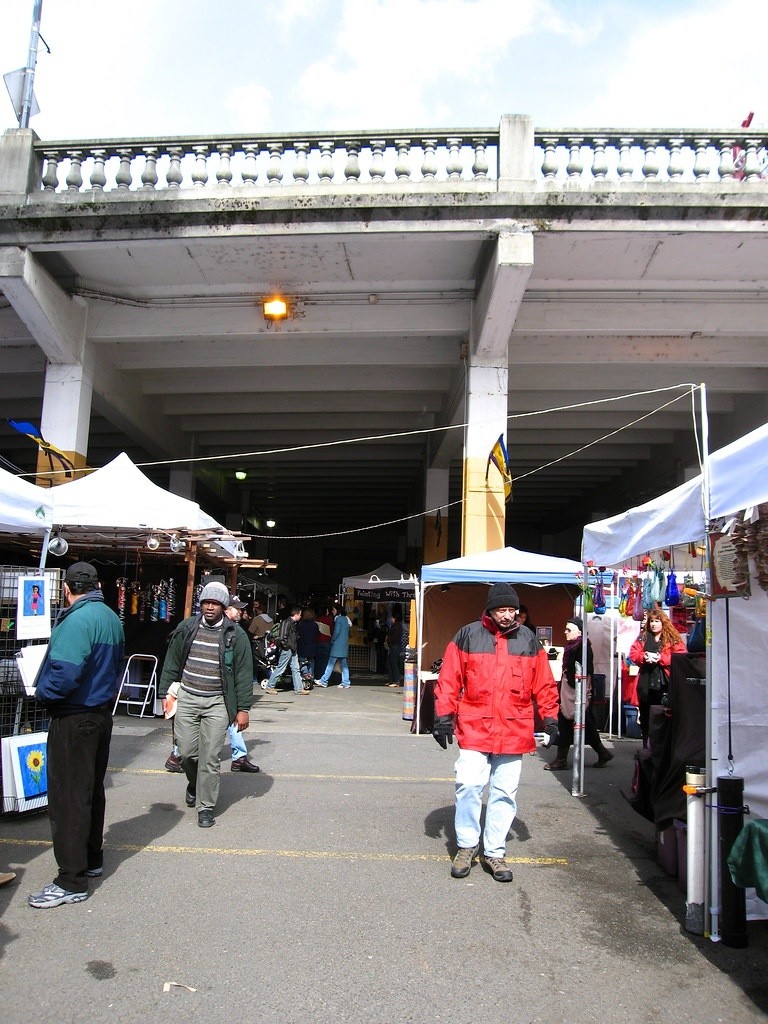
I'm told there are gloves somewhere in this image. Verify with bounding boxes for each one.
[543,719,559,749]
[432,717,452,749]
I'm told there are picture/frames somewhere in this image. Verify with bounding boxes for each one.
[11,732,49,812]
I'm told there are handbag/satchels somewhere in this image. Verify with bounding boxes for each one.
[586,559,707,653]
[650,665,671,693]
[560,677,592,721]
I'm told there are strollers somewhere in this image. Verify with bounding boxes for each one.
[260,632,313,691]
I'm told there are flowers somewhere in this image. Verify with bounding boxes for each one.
[576,559,606,584]
[622,550,672,588]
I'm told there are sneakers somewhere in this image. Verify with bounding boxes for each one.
[314,679,327,688]
[165,751,184,773]
[84,866,102,877]
[28,882,88,908]
[198,810,215,827]
[265,687,278,695]
[231,756,259,772]
[294,690,311,695]
[451,843,479,877]
[186,791,196,807]
[337,684,351,689]
[483,856,512,882]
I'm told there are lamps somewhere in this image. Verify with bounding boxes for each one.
[147,529,159,549]
[263,301,288,321]
[267,519,277,528]
[170,533,186,552]
[48,524,68,557]
[235,467,247,479]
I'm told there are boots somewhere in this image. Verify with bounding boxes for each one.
[592,740,614,767]
[545,742,569,771]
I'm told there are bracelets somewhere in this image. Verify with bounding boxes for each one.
[242,710,248,713]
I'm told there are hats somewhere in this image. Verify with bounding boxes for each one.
[199,581,229,609]
[228,594,248,608]
[566,617,583,630]
[65,561,99,582]
[488,583,519,611]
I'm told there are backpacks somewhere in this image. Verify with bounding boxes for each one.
[270,620,292,647]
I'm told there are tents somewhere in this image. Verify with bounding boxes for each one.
[0,451,249,621]
[571,420,767,942]
[416,546,615,742]
[341,562,415,606]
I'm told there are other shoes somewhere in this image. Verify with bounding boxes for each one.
[0,872,16,885]
[386,682,400,687]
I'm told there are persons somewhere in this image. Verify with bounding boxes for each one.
[27,560,125,909]
[158,580,253,829]
[385,610,403,687]
[314,602,352,689]
[431,582,616,882]
[628,609,687,747]
[369,614,387,674]
[164,593,259,774]
[242,597,321,695]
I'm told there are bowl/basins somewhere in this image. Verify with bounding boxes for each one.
[648,653,657,661]
